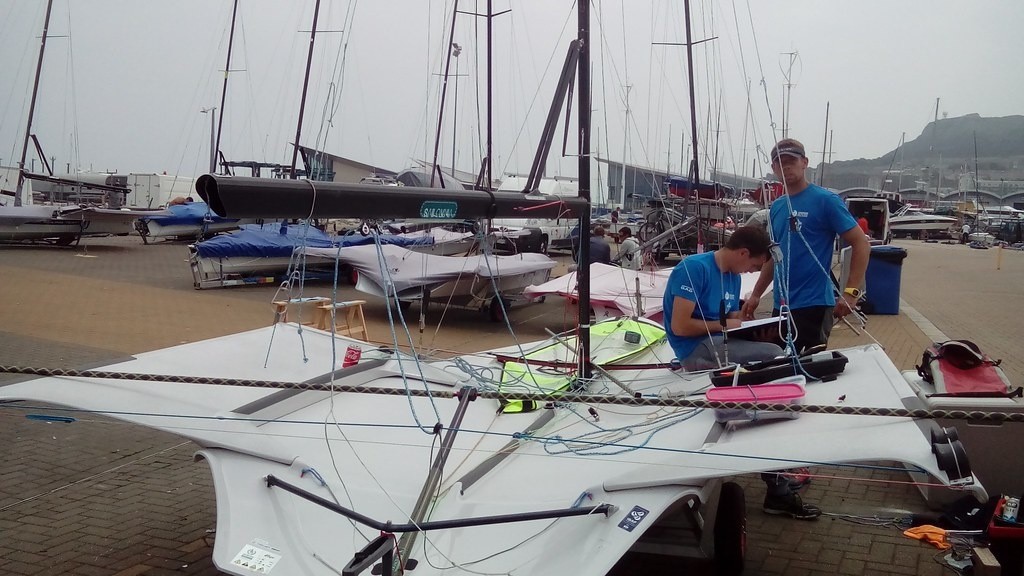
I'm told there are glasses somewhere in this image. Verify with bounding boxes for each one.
[619,235,627,237]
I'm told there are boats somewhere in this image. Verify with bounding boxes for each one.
[886,204,957,231]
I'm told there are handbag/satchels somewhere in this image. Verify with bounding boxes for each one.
[916,339,1024,398]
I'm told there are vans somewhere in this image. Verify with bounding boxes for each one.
[835,196,891,250]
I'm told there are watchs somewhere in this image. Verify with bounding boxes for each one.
[844,288,861,297]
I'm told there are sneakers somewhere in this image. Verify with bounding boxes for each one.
[788,468,812,489]
[761,491,822,518]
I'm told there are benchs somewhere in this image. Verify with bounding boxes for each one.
[273,297,330,330]
[318,299,369,342]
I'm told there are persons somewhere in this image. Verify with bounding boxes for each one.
[663,225,822,520]
[961,223,971,243]
[741,138,871,357]
[611,207,620,223]
[611,227,641,271]
[844,210,868,247]
[576,227,610,266]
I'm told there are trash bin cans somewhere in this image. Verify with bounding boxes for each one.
[862,244,908,315]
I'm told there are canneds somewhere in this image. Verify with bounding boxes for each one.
[1002,498,1019,522]
[341,344,361,368]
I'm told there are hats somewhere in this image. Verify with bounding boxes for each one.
[771,139,805,162]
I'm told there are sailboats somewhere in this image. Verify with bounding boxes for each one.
[135,0,837,333]
[0,0,991,575]
[1,1,173,248]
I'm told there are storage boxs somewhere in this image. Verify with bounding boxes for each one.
[706,383,805,421]
